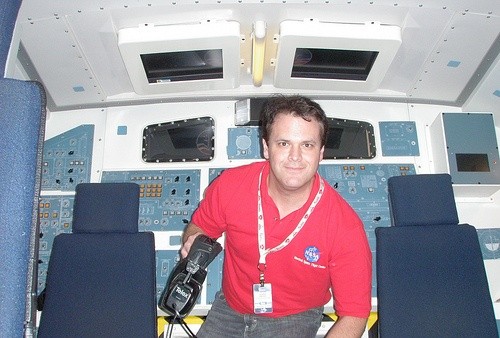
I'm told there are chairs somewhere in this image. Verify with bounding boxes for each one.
[375,172,499,338]
[37,183,158,338]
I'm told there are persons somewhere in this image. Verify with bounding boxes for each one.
[178,96,373,338]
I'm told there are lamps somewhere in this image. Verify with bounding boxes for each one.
[249,22,267,86]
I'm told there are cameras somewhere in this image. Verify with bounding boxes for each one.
[158,234,222,319]
